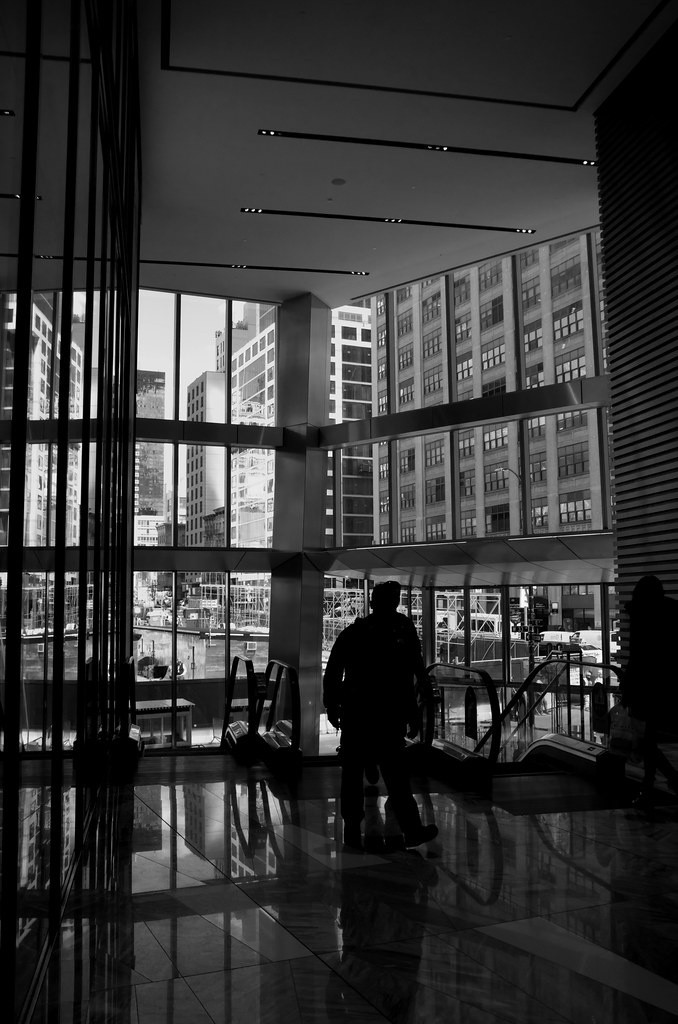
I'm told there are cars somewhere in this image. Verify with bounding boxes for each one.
[540,629,621,663]
[404,610,521,639]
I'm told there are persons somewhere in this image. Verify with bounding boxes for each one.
[584,672,592,711]
[617,574,678,808]
[536,680,550,717]
[594,669,603,684]
[323,580,438,853]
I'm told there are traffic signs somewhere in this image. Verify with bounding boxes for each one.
[526,633,540,642]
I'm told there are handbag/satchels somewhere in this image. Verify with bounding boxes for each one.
[610,704,645,766]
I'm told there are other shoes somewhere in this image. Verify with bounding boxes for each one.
[405,824,438,847]
[669,771,678,793]
[632,792,656,811]
[344,828,362,841]
